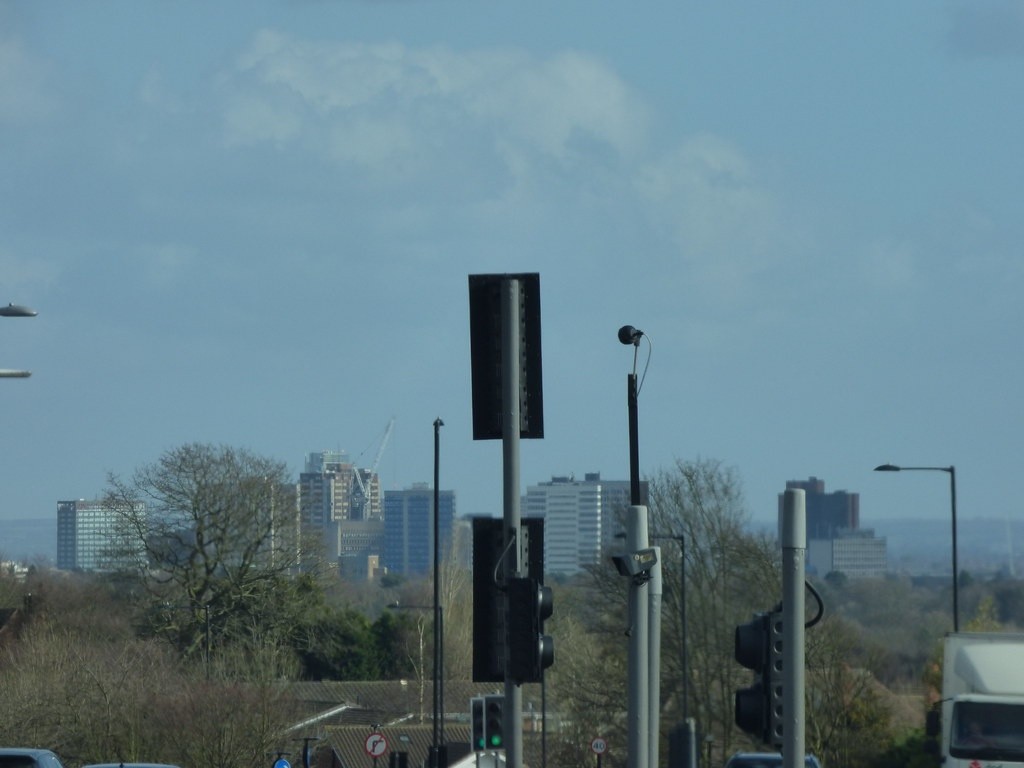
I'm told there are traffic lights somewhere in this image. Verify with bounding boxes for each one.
[483,694,505,751]
[733,605,789,750]
[471,697,486,754]
[509,578,555,690]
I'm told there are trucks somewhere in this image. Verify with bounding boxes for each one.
[924,632,1024,768]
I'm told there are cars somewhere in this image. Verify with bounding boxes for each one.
[0,748,65,768]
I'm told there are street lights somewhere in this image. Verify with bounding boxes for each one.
[432,418,450,768]
[613,324,669,767]
[613,532,698,768]
[875,463,959,632]
[386,601,444,746]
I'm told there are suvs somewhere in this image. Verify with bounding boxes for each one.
[726,752,820,768]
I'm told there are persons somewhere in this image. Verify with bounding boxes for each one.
[958,714,997,747]
